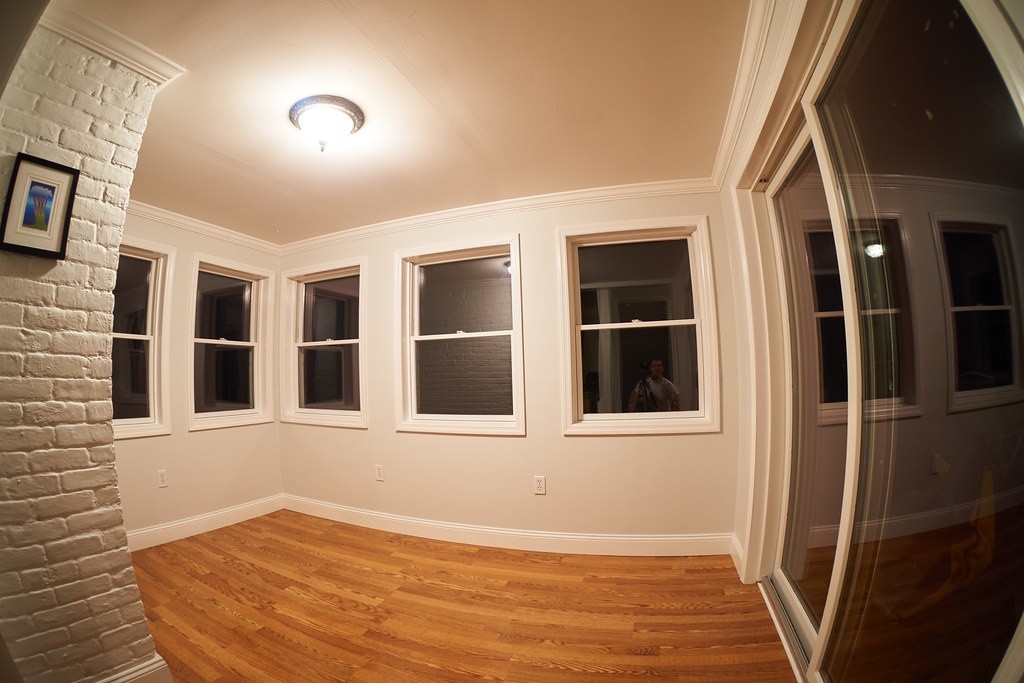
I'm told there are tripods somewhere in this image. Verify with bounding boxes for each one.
[631,379,657,412]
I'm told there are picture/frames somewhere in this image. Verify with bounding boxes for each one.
[0,151,81,261]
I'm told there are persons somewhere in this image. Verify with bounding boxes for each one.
[626,357,681,412]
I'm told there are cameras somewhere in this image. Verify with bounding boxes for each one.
[638,362,648,378]
